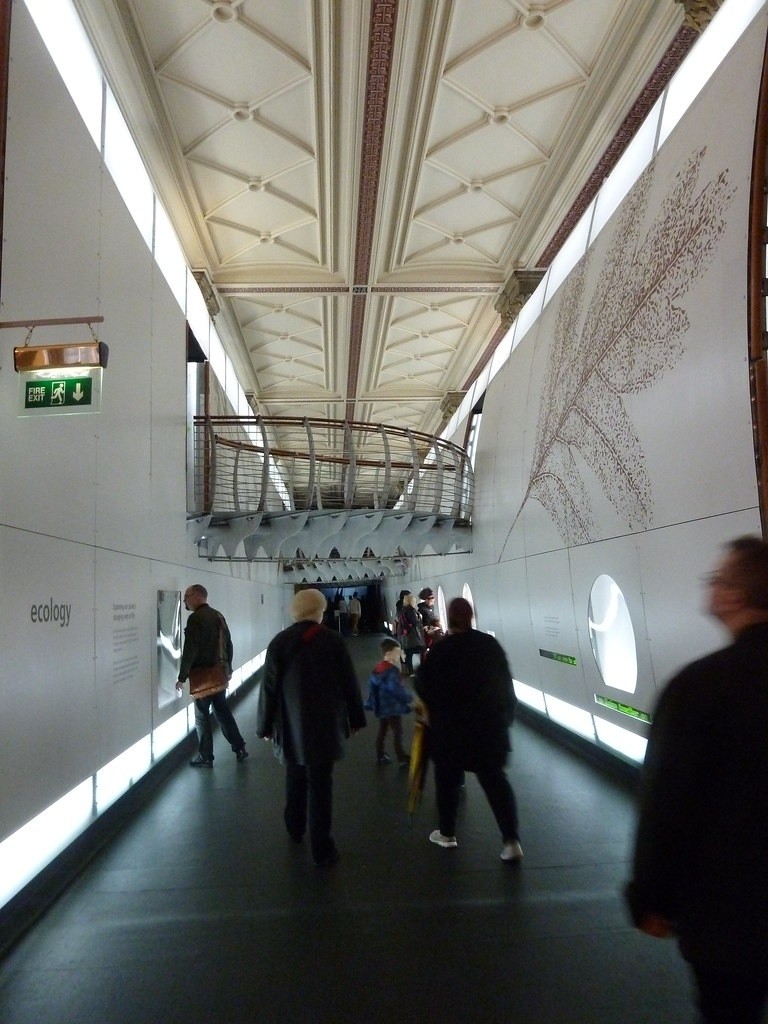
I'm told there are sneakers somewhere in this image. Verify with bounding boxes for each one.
[500,841,523,860]
[429,830,457,848]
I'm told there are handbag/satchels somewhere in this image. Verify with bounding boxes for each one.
[188,663,227,699]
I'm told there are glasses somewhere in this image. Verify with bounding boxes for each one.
[703,569,744,589]
[426,597,434,600]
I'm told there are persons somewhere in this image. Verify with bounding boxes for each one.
[174,585,250,770]
[362,637,416,766]
[410,599,525,863]
[392,588,442,677]
[254,587,368,865]
[623,536,768,1024]
[319,591,362,636]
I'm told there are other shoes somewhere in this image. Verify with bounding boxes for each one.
[376,752,392,762]
[400,649,406,663]
[236,749,248,760]
[396,753,410,761]
[314,848,339,865]
[190,756,214,768]
[409,671,416,677]
